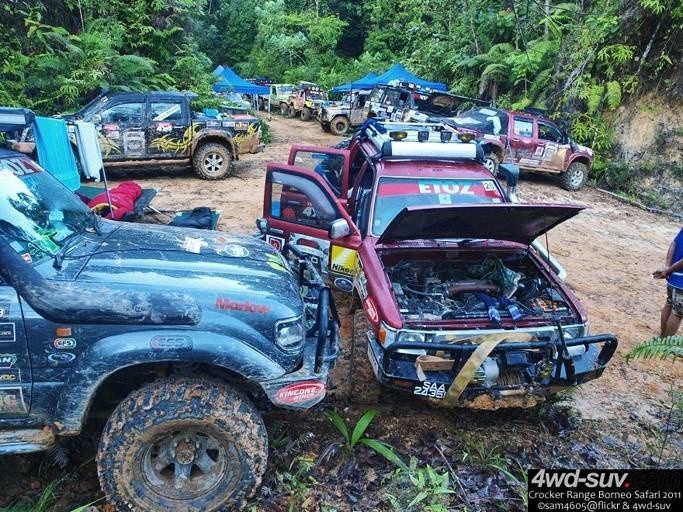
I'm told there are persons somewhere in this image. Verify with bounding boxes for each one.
[652,227,683,339]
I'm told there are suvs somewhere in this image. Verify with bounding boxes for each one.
[239,78,330,123]
[253,112,621,418]
[0,115,347,512]
[406,100,598,192]
[322,82,467,137]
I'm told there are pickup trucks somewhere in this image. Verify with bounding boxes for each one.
[4,90,266,181]
